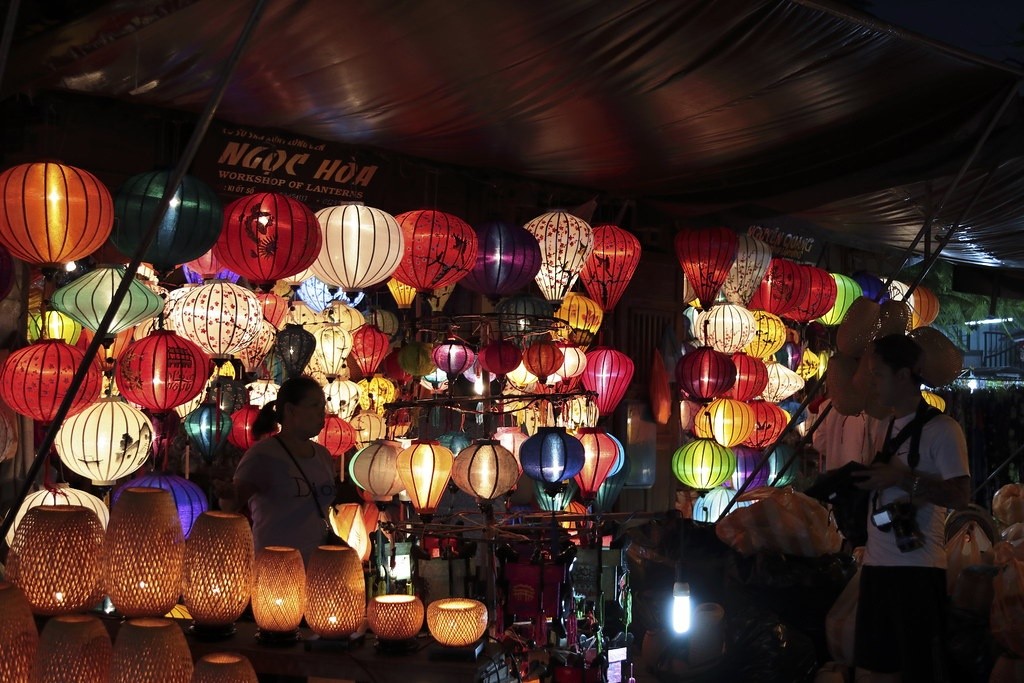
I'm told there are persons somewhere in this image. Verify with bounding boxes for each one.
[229,374,340,556]
[861,335,970,682]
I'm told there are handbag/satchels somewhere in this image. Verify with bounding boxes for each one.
[944,483,1024,660]
[826,547,865,668]
[716,484,842,556]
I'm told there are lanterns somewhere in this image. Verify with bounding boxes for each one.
[0,146,941,682]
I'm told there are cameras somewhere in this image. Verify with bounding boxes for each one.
[871,501,925,554]
